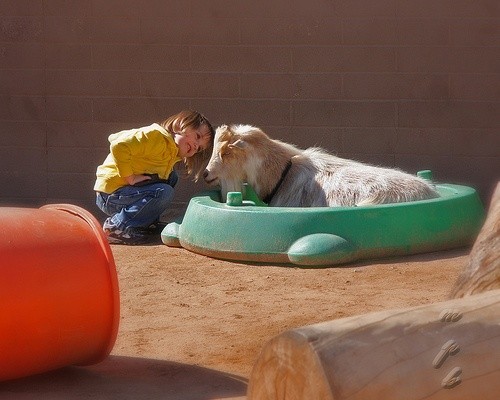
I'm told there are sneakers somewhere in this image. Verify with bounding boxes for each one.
[142,222,169,235]
[103,217,151,245]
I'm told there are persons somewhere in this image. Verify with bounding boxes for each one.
[93,111,215,245]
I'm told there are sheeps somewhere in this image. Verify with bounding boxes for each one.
[204,121,440,208]
